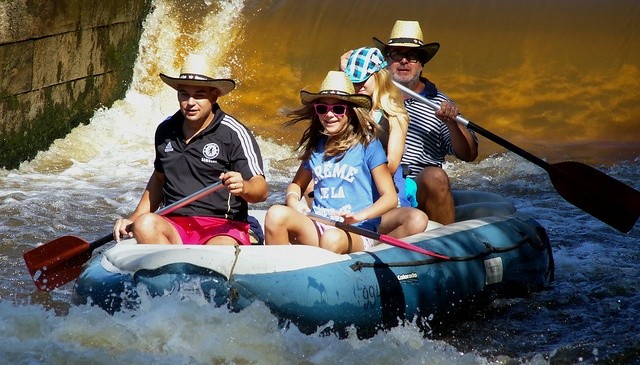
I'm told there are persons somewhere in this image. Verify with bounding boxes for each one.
[374,21,478,224]
[113,56,268,245]
[337,46,409,172]
[265,71,398,253]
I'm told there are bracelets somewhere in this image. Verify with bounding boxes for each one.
[285,192,300,201]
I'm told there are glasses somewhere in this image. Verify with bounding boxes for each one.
[388,49,420,63]
[311,102,347,117]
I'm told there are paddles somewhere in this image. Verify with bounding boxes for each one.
[306,212,492,261]
[23,179,227,292]
[389,78,639,236]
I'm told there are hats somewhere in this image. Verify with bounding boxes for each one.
[344,46,387,83]
[374,20,440,64]
[159,53,236,96]
[300,70,373,113]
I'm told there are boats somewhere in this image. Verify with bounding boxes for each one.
[73,188,555,338]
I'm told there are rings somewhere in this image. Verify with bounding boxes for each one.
[235,183,238,190]
[451,106,455,109]
